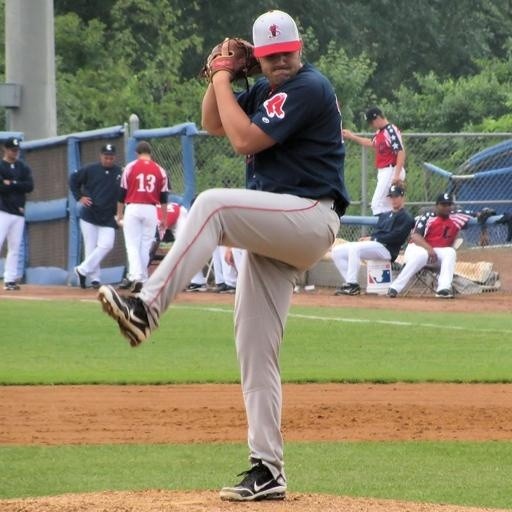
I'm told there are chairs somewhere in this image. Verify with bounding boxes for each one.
[404,237,464,298]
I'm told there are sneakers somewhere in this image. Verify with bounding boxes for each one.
[95,283,153,346]
[219,463,287,502]
[184,282,236,294]
[119,279,143,291]
[333,283,361,296]
[2,281,21,291]
[435,289,454,299]
[387,288,397,297]
[74,265,86,289]
[91,280,100,288]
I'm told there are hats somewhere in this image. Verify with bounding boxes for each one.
[4,136,23,151]
[99,144,116,156]
[363,107,382,122]
[437,192,455,206]
[385,184,405,198]
[250,9,302,58]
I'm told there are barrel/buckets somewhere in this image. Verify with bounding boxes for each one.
[366,259,392,294]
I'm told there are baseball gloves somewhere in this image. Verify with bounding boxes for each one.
[201,37,261,81]
[478,207,496,224]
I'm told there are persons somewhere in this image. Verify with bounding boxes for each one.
[341,107,407,220]
[0,137,33,290]
[96,10,351,503]
[387,192,489,299]
[69,141,243,294]
[331,184,414,297]
